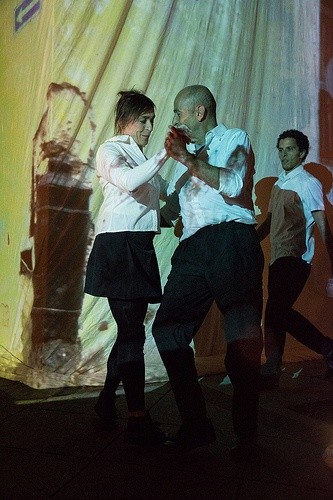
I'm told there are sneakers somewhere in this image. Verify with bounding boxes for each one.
[94,390,119,431]
[127,416,167,446]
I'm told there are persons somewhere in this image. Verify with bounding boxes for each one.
[85,90,193,441]
[151,85,265,465]
[256,130,333,386]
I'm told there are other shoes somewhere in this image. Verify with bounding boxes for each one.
[232,432,257,459]
[258,358,282,377]
[165,417,217,450]
[326,340,333,371]
